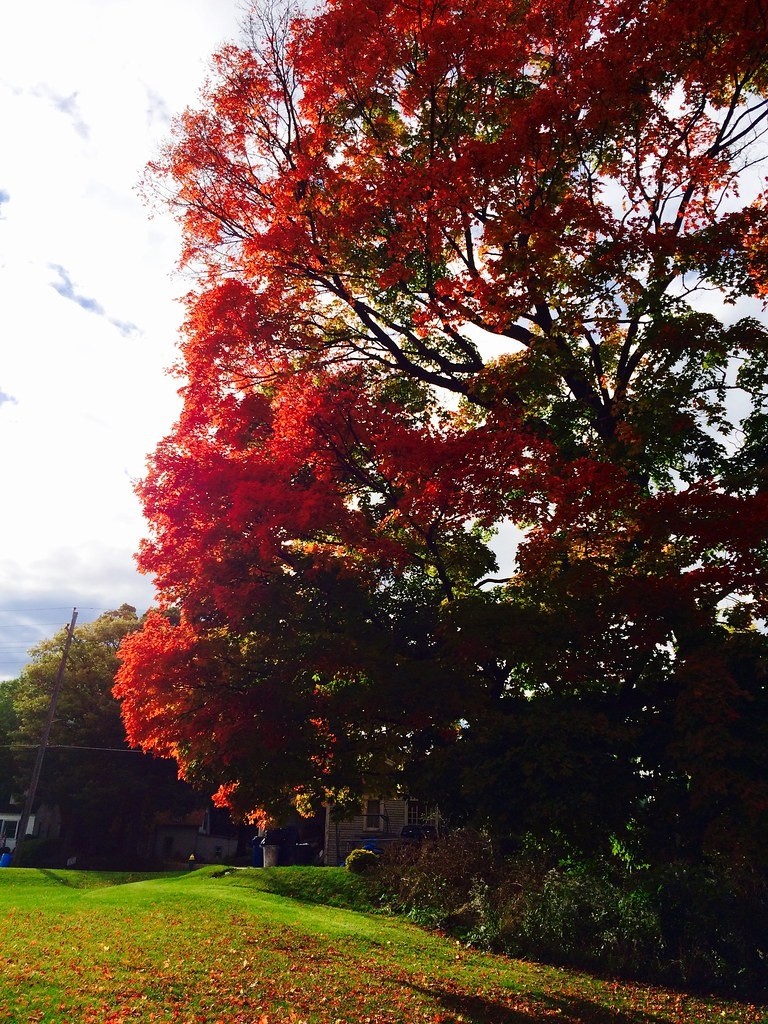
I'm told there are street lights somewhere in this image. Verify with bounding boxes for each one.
[12,719,80,868]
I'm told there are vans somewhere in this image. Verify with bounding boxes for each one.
[397,825,442,853]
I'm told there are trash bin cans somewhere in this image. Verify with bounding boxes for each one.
[251,836,316,868]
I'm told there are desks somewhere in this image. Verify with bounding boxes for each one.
[340,838,399,864]
